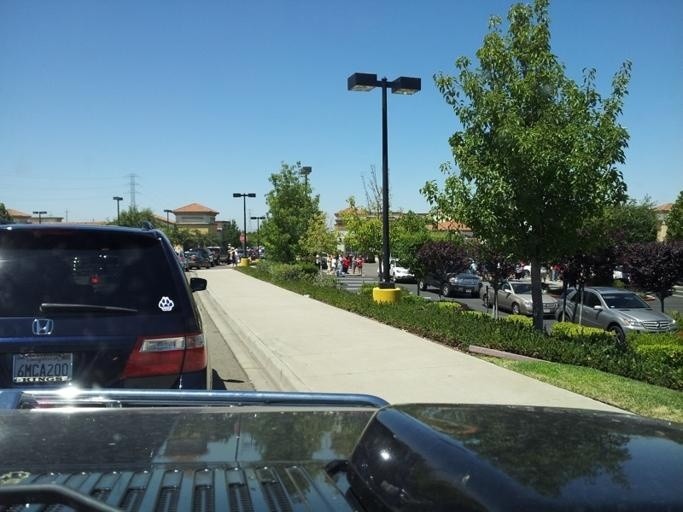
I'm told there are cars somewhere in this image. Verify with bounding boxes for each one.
[555,286,678,343]
[0,221,212,389]
[376,259,415,284]
[209,246,231,265]
[419,263,481,296]
[479,279,558,316]
[0,383,682,512]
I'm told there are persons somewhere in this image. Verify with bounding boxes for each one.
[324,252,363,276]
[477,262,485,276]
[227,247,235,266]
[233,246,240,264]
[468,260,477,274]
[513,261,562,282]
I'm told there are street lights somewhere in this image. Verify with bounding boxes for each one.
[31,210,47,222]
[234,192,255,258]
[253,216,265,258]
[349,73,421,290]
